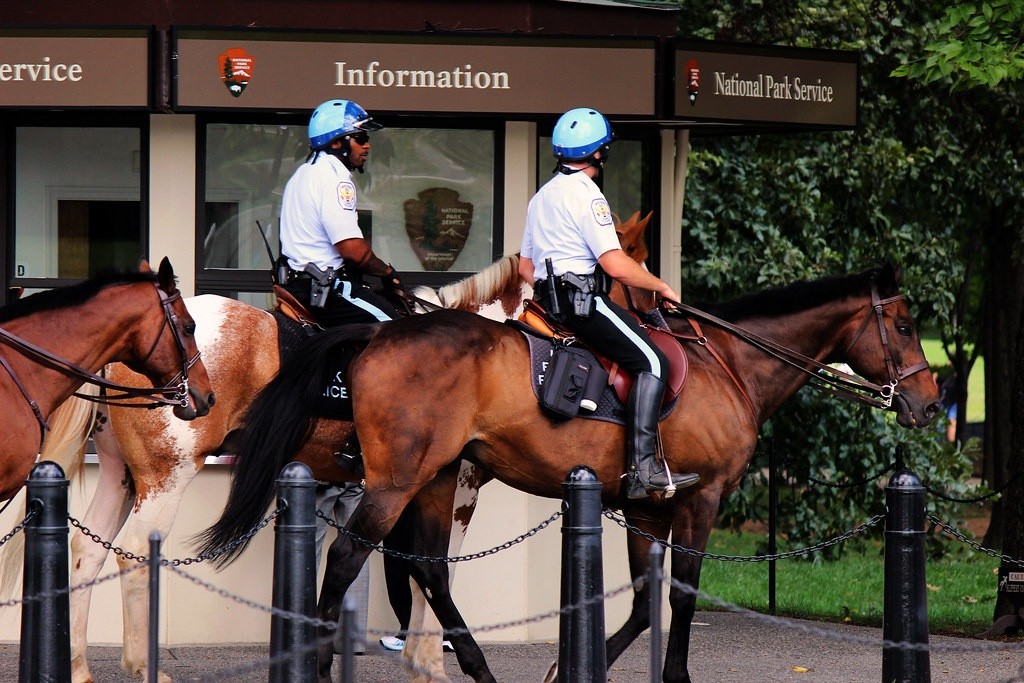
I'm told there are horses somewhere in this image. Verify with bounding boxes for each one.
[0,210,656,683]
[185,260,940,683]
[0,256,216,503]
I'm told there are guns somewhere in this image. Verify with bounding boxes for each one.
[305,262,334,308]
[562,271,597,317]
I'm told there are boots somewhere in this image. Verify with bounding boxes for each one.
[331,435,366,479]
[621,372,701,499]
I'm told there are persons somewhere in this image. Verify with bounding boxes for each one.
[518,106,700,500]
[929,373,960,542]
[272,98,411,462]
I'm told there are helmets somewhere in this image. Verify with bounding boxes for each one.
[308,98,384,151]
[552,107,615,161]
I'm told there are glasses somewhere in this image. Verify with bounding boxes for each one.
[351,134,371,146]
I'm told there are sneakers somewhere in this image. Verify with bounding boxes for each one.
[442,640,455,652]
[379,636,405,652]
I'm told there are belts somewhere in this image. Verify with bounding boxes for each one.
[288,271,343,281]
